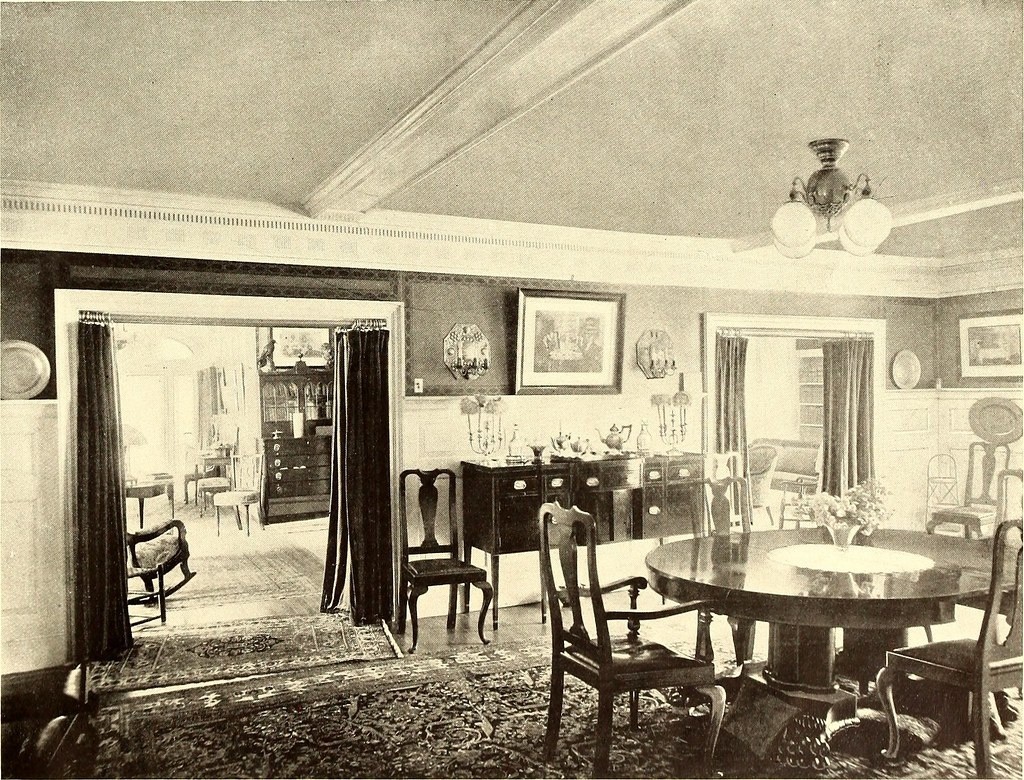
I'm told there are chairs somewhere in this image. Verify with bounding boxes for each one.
[124,483,196,625]
[213,452,266,538]
[777,475,821,531]
[535,498,727,779]
[397,468,495,653]
[121,424,174,529]
[747,444,778,525]
[875,517,1022,779]
[687,475,750,539]
[926,441,1011,540]
[199,443,238,518]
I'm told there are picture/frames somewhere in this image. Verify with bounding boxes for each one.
[956,308,1023,383]
[513,286,625,396]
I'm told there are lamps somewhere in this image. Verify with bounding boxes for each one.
[769,137,892,259]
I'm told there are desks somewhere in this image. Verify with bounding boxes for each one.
[645,524,1019,726]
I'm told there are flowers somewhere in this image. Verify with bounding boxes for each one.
[811,478,895,536]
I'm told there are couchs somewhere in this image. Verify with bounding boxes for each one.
[752,438,823,493]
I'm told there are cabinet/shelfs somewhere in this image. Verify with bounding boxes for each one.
[256,367,334,522]
[461,451,708,629]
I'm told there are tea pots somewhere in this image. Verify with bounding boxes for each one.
[594,423,631,452]
[508,423,527,457]
[550,431,590,453]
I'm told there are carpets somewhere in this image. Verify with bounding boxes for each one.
[85,547,1021,778]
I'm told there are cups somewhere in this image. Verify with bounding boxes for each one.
[528,444,546,464]
[936,378,942,388]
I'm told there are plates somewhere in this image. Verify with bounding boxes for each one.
[1,340,51,400]
[893,349,921,389]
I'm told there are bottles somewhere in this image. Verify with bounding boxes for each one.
[637,424,654,457]
